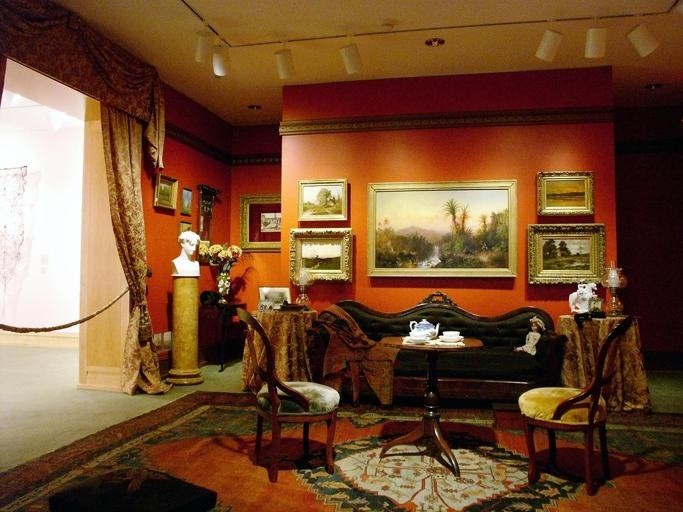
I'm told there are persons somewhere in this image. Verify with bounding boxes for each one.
[172,230,201,276]
[514,316,547,356]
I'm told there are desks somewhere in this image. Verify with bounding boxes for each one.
[206,302,248,373]
[380,338,484,477]
[242,311,318,391]
[556,315,652,414]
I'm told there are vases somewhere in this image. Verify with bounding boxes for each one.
[217,274,230,304]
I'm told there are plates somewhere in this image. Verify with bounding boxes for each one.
[403,337,428,345]
[439,336,464,342]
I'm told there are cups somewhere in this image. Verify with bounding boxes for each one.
[263,302,273,312]
[258,301,266,311]
[443,331,461,338]
[409,332,426,339]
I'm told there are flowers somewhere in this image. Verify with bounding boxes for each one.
[199,245,241,273]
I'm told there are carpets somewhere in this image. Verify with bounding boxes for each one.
[0,392,683,512]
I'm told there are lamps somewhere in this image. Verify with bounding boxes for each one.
[296,270,311,305]
[605,261,624,316]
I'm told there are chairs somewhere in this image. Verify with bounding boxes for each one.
[236,308,341,482]
[518,317,636,495]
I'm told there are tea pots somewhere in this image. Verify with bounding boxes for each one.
[410,319,440,340]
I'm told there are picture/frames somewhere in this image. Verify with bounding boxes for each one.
[537,171,593,215]
[290,228,353,284]
[178,221,192,233]
[367,180,516,278]
[528,225,605,283]
[298,179,348,222]
[153,174,179,210]
[180,187,192,216]
[239,195,281,252]
[258,285,291,310]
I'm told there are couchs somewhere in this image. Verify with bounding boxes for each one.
[309,291,568,399]
